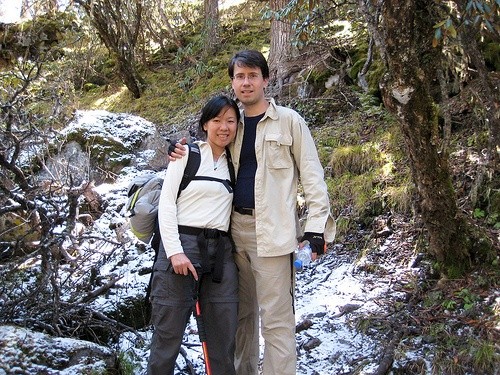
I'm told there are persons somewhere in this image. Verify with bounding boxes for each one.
[168,50,337,374]
[148,96,239,375]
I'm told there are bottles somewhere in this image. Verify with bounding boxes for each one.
[294,243,313,269]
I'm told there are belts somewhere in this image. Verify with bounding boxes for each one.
[233,206,254,216]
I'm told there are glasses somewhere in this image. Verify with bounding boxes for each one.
[231,73,264,79]
